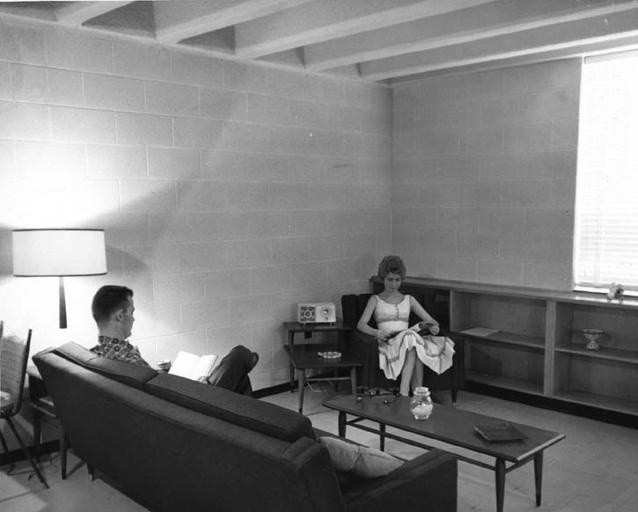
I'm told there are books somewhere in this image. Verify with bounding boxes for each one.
[385,322,437,341]
[473,422,527,444]
[168,352,219,383]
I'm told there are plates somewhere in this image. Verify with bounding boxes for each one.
[318,351,342,358]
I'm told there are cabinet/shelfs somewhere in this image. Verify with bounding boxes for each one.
[367,275,638,420]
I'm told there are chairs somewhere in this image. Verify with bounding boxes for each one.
[0,322,49,491]
[341,293,457,407]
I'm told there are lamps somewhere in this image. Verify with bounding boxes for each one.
[10,229,107,346]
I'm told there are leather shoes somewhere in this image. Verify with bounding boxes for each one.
[248,353,258,372]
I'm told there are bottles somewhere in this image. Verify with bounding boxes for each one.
[410,387,434,420]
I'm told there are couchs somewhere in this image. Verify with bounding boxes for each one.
[32,340,457,512]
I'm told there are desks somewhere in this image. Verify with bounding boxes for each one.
[283,323,364,416]
[26,369,90,479]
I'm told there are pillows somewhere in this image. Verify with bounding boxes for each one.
[317,436,404,478]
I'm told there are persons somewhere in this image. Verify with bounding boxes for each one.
[89,285,260,399]
[357,256,441,397]
